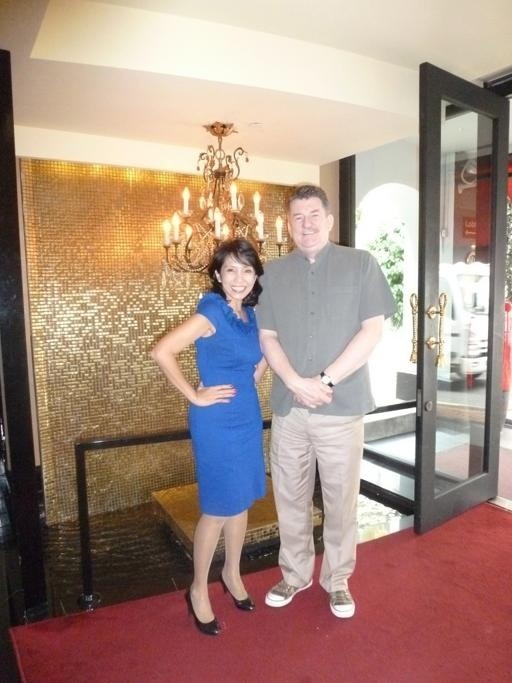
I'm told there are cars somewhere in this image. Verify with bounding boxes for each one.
[446,292,489,383]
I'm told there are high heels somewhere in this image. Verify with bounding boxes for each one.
[185,586,222,637]
[219,569,257,612]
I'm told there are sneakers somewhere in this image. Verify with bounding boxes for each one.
[264,576,314,610]
[327,589,356,620]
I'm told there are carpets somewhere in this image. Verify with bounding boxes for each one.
[435,443,511,498]
[6,503,511,683]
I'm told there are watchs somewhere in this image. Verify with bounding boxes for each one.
[318,369,334,390]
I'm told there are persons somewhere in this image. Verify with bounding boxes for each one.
[251,182,402,622]
[151,235,272,637]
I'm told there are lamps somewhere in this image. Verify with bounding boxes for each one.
[158,119,289,277]
[456,160,481,195]
